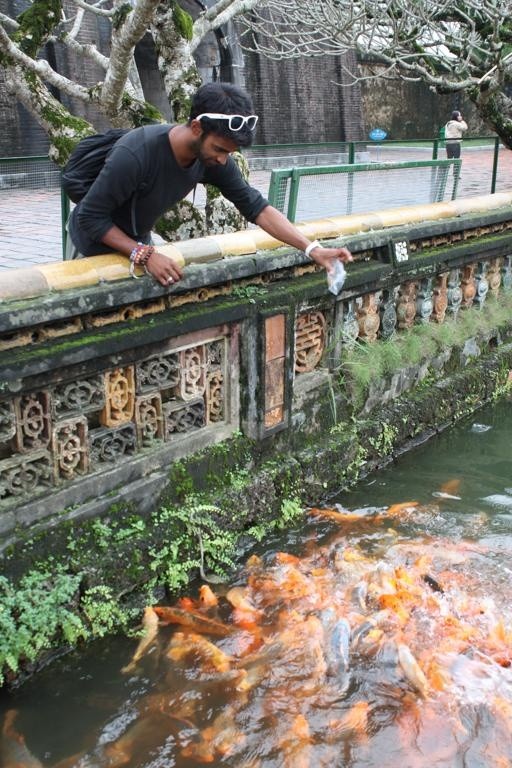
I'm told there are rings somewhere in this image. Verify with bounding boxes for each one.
[167,277,173,282]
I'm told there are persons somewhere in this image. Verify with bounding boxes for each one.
[65,83,354,291]
[444,111,468,178]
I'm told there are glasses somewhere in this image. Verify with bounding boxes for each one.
[195,112,260,132]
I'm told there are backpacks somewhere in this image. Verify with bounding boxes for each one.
[60,125,161,205]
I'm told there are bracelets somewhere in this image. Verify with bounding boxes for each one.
[140,246,155,265]
[304,240,323,259]
[130,242,146,263]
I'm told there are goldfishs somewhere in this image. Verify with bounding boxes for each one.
[51,501,512,768]
